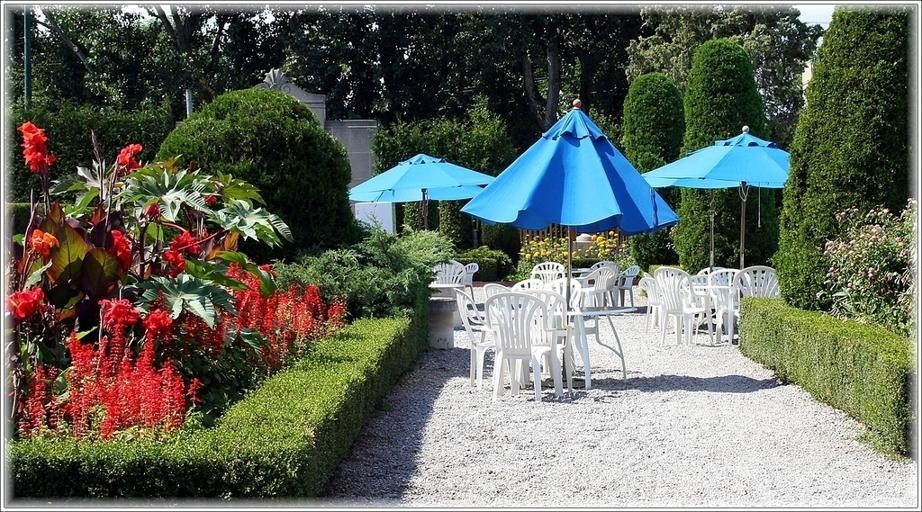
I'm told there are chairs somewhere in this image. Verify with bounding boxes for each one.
[639,265,779,350]
[433,258,485,327]
[451,258,639,403]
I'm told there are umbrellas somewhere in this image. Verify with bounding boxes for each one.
[642,126,791,270]
[459,98,680,381]
[348,152,495,228]
